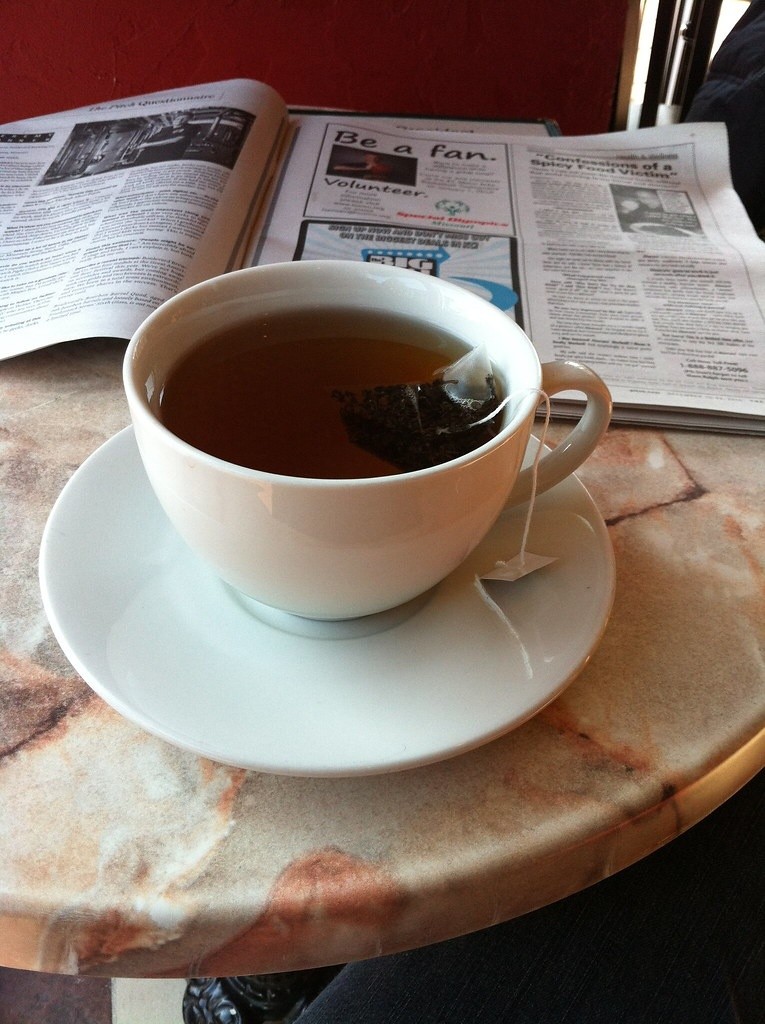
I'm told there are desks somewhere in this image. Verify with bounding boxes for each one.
[0,106,765,1024]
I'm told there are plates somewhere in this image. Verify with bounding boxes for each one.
[38,420,616,777]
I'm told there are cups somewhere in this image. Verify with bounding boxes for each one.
[122,259,612,620]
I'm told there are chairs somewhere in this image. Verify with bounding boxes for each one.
[0,0,641,134]
[292,774,765,1024]
